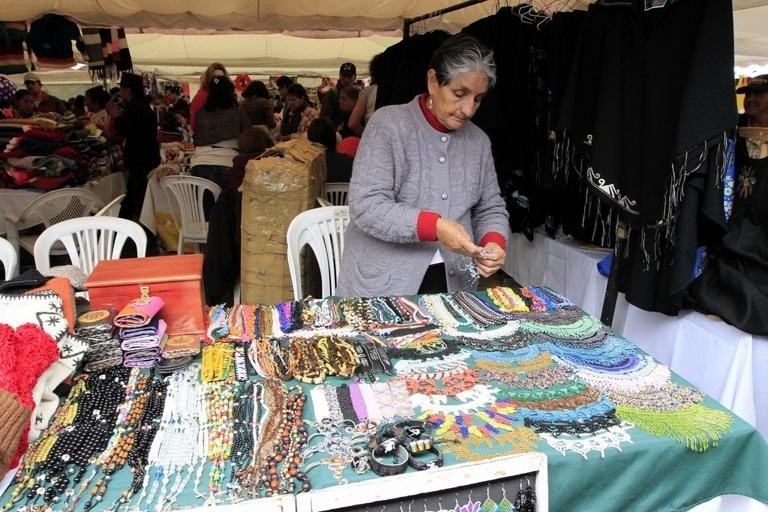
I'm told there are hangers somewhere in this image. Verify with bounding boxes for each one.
[402,0,672,41]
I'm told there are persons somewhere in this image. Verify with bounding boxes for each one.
[189,62,238,144]
[273,76,296,112]
[274,85,319,143]
[84,84,112,128]
[1,90,39,121]
[66,94,84,115]
[335,34,511,291]
[22,70,68,116]
[348,52,396,135]
[337,86,363,136]
[165,83,191,132]
[163,112,189,144]
[308,118,353,183]
[176,110,194,142]
[238,81,280,130]
[319,61,356,132]
[107,70,159,219]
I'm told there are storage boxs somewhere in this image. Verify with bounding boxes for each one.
[84,254,207,336]
[240,139,328,304]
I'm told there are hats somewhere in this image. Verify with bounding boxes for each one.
[23,72,42,85]
[735,74,766,97]
[339,62,356,77]
[116,72,145,90]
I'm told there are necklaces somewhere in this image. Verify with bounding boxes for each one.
[200,293,448,381]
[439,428,538,460]
[497,386,734,453]
[403,367,478,395]
[421,401,518,437]
[422,286,575,321]
[458,315,636,364]
[471,351,670,394]
[1,362,309,512]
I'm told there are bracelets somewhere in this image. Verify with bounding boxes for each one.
[367,418,444,477]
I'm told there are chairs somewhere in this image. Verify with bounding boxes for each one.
[1,237,18,282]
[286,205,357,300]
[95,193,127,217]
[324,182,351,206]
[316,197,334,207]
[159,175,222,255]
[5,187,107,257]
[34,216,147,291]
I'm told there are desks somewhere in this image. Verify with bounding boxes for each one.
[1,169,131,262]
[0,285,762,511]
